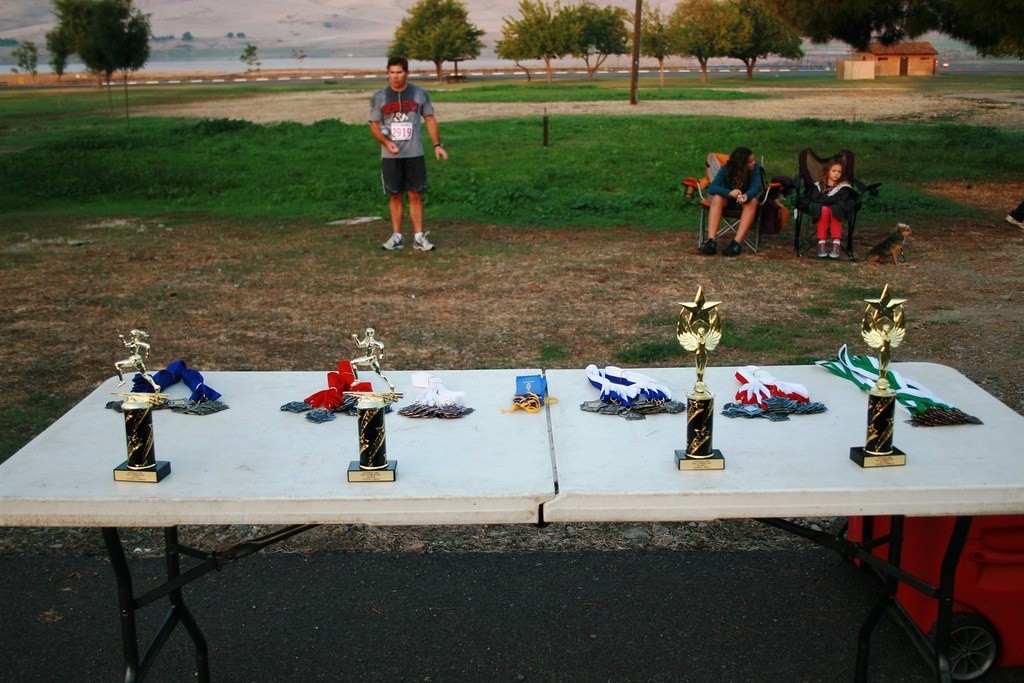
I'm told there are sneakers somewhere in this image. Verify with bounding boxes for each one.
[412,230,435,251]
[382,232,404,250]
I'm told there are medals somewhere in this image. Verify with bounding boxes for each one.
[579,398,685,421]
[720,395,828,421]
[280,401,313,413]
[152,397,229,416]
[397,404,476,419]
[334,396,393,416]
[105,400,124,413]
[305,409,336,423]
[904,407,984,427]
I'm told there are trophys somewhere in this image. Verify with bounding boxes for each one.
[342,328,405,482]
[849,283,909,468]
[674,287,726,471]
[110,329,171,483]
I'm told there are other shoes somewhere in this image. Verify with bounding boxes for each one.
[701,238,717,255]
[722,239,742,256]
[1005,209,1024,229]
[817,240,828,257]
[829,239,840,258]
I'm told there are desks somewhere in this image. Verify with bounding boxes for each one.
[0,363,1024,683]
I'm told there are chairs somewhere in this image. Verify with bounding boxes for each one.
[788,147,882,260]
[681,152,783,255]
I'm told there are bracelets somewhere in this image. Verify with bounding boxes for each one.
[434,144,443,148]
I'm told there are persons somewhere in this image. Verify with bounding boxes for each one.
[367,57,448,252]
[700,147,761,257]
[808,161,859,259]
[1005,200,1024,230]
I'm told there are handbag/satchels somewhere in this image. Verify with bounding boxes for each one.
[752,200,782,234]
[776,199,789,228]
